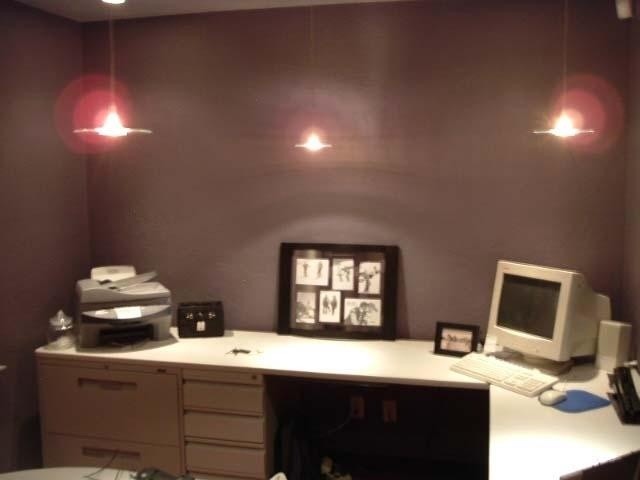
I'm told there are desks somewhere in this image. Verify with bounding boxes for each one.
[31,325,640,480]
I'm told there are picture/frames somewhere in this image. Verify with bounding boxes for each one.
[432,320,480,359]
[275,239,403,343]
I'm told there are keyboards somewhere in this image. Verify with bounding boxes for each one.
[453,352,559,396]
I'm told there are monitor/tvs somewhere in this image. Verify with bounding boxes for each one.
[486,260,612,374]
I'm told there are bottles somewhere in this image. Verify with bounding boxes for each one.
[47,309,73,349]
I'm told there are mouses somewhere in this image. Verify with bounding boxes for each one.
[540,389,567,405]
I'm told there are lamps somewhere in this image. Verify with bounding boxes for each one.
[529,0,597,140]
[70,1,156,141]
[294,4,335,155]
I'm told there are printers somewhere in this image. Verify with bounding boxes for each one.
[75,267,172,347]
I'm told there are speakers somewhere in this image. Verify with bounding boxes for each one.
[595,319,632,373]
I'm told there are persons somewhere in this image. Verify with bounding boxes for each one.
[298,260,377,326]
[445,334,457,351]
